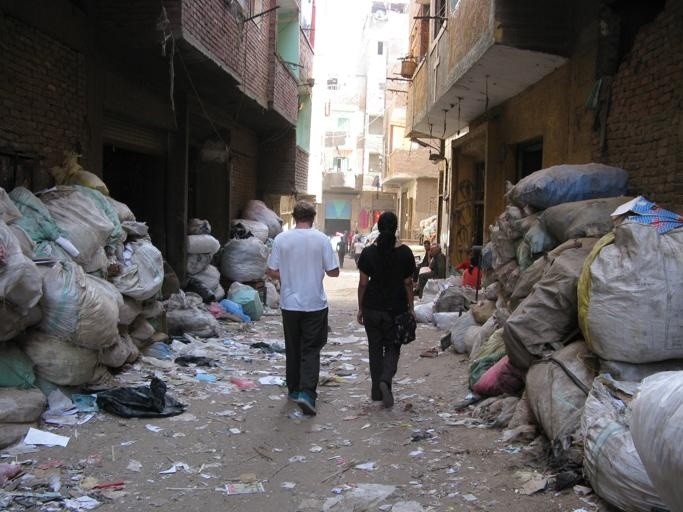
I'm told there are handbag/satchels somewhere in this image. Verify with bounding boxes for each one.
[393,311,416,345]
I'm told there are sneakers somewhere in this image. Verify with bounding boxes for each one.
[378,379,394,408]
[296,391,317,416]
[287,391,299,403]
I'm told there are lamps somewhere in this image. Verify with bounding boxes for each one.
[429,154,449,201]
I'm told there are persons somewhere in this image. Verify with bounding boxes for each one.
[459,249,483,290]
[263,200,340,417]
[355,210,419,409]
[410,240,432,287]
[419,331,452,360]
[327,228,371,269]
[452,250,472,274]
[412,243,445,299]
